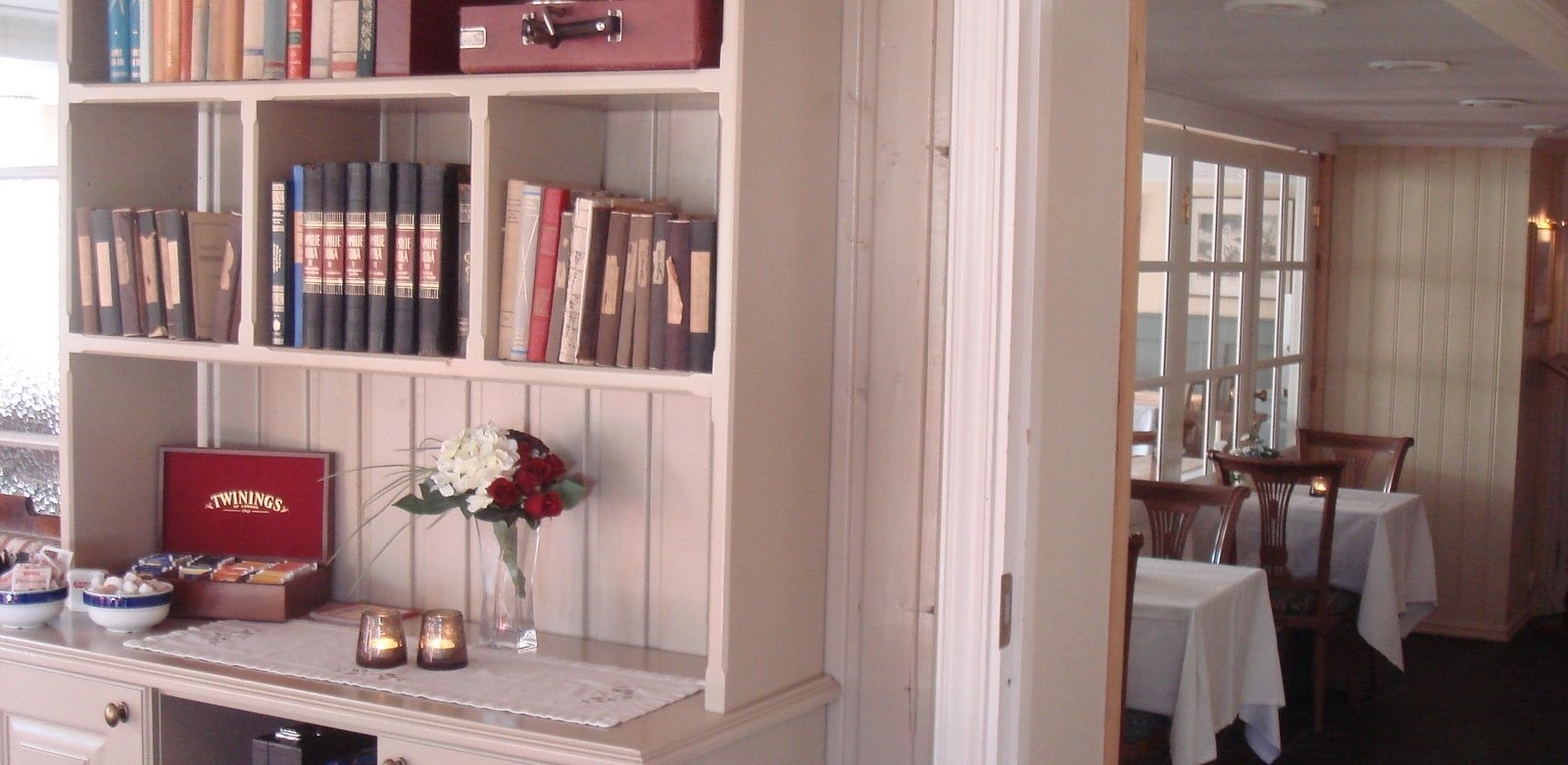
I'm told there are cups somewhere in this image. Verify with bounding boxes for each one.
[416,608,468,671]
[356,609,407,669]
[1309,475,1332,497]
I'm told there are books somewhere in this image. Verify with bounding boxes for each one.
[266,159,471,358]
[74,204,242,346]
[104,1,414,84]
[495,177,719,375]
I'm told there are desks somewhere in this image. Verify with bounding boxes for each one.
[1130,553,1274,741]
[1236,479,1440,695]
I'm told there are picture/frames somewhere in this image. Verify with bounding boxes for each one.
[1188,194,1297,303]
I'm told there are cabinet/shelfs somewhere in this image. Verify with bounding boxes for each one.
[0,0,843,765]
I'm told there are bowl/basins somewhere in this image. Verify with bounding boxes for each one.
[82,580,174,635]
[0,578,69,630]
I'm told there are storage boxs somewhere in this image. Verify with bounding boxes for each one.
[128,438,337,626]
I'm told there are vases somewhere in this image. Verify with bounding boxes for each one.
[475,522,542,657]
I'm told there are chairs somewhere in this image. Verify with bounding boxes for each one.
[1291,426,1417,494]
[1207,449,1369,743]
[1129,472,1244,574]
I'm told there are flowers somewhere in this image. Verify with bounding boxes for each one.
[396,422,594,527]
